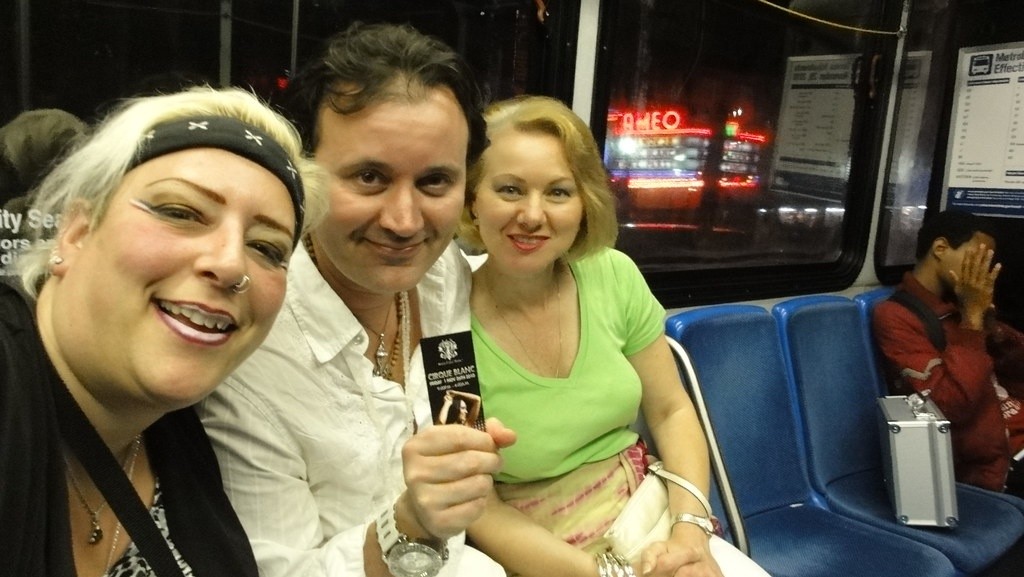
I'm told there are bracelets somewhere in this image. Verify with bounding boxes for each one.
[598,552,634,577]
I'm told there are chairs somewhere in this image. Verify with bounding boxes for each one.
[770,293,1023,576]
[851,290,1024,513]
[666,304,955,576]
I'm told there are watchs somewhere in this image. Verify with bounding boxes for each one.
[670,514,715,537]
[375,505,449,577]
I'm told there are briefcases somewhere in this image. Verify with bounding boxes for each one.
[876,389,960,529]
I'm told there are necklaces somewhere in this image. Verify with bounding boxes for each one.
[306,233,404,380]
[490,281,563,378]
[63,439,140,577]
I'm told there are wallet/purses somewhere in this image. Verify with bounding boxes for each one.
[605,462,718,576]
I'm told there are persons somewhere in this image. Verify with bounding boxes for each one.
[872,209,1024,499]
[194,21,517,577]
[428,95,770,577]
[437,389,481,428]
[0,85,329,577]
